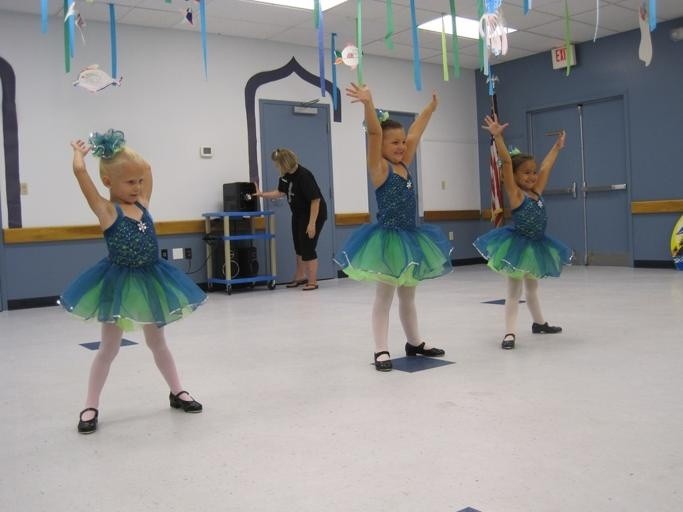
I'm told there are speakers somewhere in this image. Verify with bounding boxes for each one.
[245,247,259,276]
[223,182,256,212]
[212,241,245,280]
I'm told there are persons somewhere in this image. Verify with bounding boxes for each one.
[331,81,455,371]
[473,109,575,350]
[251,148,328,291]
[56,127,210,434]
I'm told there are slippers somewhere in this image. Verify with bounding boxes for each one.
[287,279,319,291]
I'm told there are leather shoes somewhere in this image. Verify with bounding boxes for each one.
[374,351,392,371]
[77,408,99,433]
[501,333,516,349]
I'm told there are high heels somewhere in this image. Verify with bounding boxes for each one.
[532,322,562,334]
[169,390,202,413]
[405,342,445,357]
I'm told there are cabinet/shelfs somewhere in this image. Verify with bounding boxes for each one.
[201,211,279,294]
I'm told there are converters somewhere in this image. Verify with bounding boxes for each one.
[186,249,191,257]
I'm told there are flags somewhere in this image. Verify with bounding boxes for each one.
[488,91,505,227]
